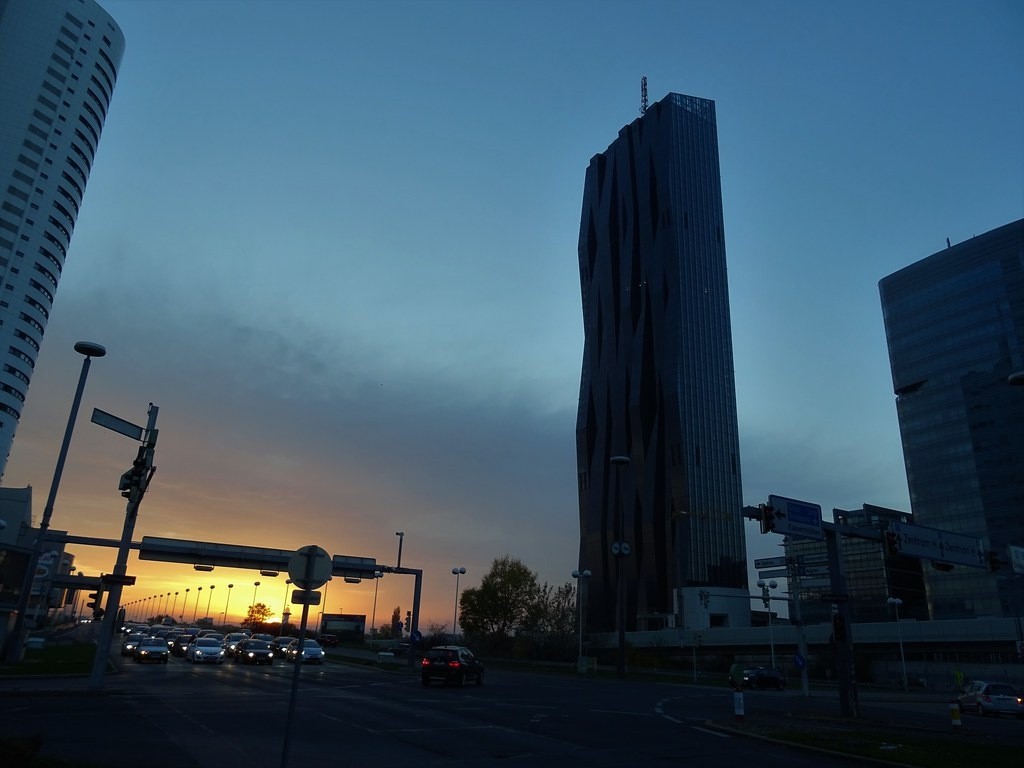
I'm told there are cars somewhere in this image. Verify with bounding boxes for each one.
[728,666,787,690]
[122,622,170,664]
[250,633,325,665]
[956,681,1024,720]
[149,625,273,665]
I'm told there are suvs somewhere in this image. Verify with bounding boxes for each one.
[421,645,484,688]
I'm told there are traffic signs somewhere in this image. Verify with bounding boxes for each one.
[769,495,824,540]
[1010,545,1024,574]
[891,521,987,569]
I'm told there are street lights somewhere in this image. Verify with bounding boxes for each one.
[572,570,592,665]
[8,342,106,663]
[609,455,629,678]
[122,580,293,636]
[757,580,775,668]
[372,573,383,629]
[452,567,466,634]
[887,598,908,689]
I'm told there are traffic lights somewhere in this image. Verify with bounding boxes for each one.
[885,530,898,555]
[987,550,1001,572]
[762,504,776,534]
